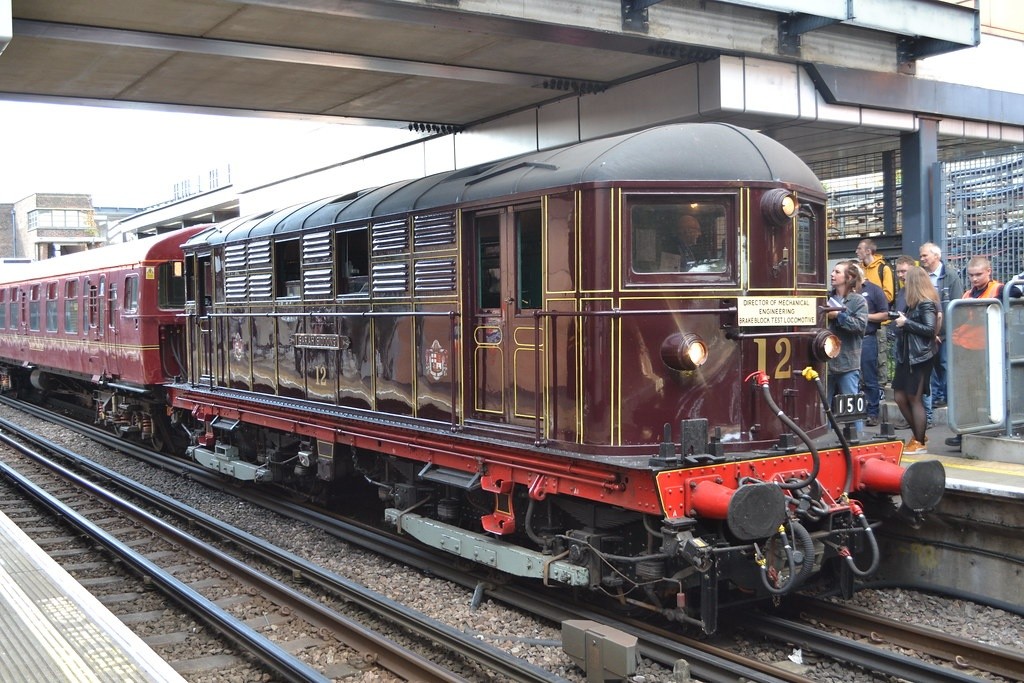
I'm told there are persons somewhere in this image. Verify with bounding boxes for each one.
[945,256,1021,446]
[893,254,942,432]
[919,243,964,408]
[893,265,938,454]
[662,214,701,273]
[828,261,868,441]
[855,239,894,400]
[851,262,888,426]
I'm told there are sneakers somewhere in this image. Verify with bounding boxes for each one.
[902,439,927,454]
[906,435,929,447]
[945,434,962,446]
[894,419,911,429]
[865,417,878,426]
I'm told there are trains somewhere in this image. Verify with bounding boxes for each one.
[0,121,951,636]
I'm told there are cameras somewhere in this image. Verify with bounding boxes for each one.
[888,312,906,320]
